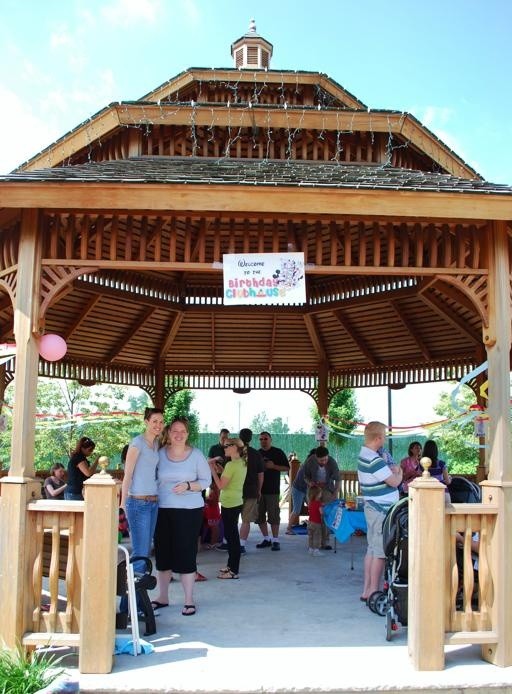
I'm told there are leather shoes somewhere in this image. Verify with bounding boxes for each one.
[256,539,271,549]
[196,572,207,581]
[240,546,246,555]
[271,542,280,550]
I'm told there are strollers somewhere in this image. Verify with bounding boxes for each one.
[383,496,411,641]
[368,476,482,617]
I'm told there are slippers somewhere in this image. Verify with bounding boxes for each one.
[360,597,368,602]
[151,601,168,609]
[366,599,376,605]
[182,604,196,615]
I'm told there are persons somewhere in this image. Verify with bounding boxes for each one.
[357,421,404,606]
[43,462,68,500]
[161,425,207,581]
[455,532,479,613]
[206,428,231,520]
[63,437,102,500]
[400,441,423,499]
[415,440,453,505]
[254,431,291,551]
[207,438,249,580]
[303,445,343,550]
[306,485,325,557]
[118,407,165,622]
[215,428,265,556]
[284,447,316,535]
[151,416,213,616]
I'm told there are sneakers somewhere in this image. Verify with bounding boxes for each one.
[312,553,324,556]
[308,551,313,554]
[216,543,228,551]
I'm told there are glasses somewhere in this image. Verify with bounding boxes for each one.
[259,438,268,441]
[224,443,234,448]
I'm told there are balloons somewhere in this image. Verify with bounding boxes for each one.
[38,333,68,362]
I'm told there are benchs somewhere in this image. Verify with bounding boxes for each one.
[42,531,157,637]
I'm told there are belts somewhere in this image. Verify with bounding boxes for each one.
[128,495,159,502]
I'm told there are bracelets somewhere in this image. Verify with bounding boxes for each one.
[186,482,191,490]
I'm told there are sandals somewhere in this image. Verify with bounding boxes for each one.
[220,567,230,572]
[217,571,239,579]
